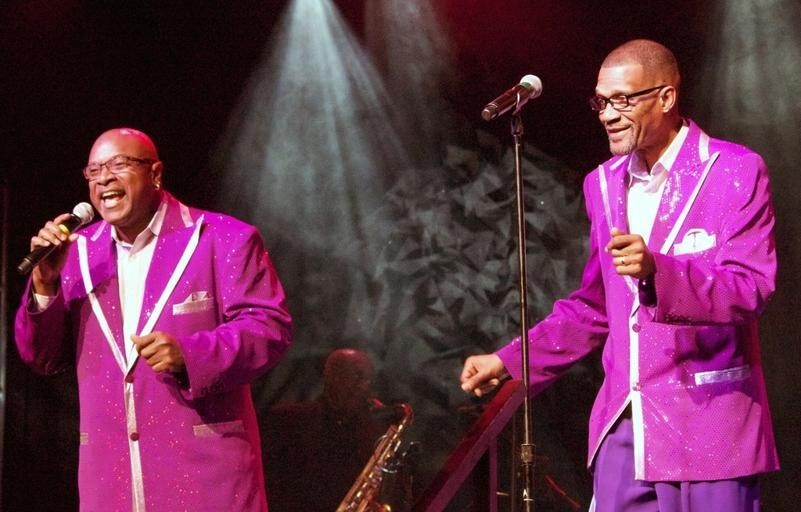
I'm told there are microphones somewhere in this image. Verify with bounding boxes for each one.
[16,199,97,280]
[480,72,547,122]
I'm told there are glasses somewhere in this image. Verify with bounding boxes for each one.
[82,156,152,180]
[588,85,667,112]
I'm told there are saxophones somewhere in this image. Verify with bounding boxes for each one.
[335,398,415,512]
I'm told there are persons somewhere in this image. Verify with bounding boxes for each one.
[459,38,781,511]
[256,346,415,511]
[12,127,296,512]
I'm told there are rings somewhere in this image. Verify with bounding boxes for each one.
[620,257,626,264]
